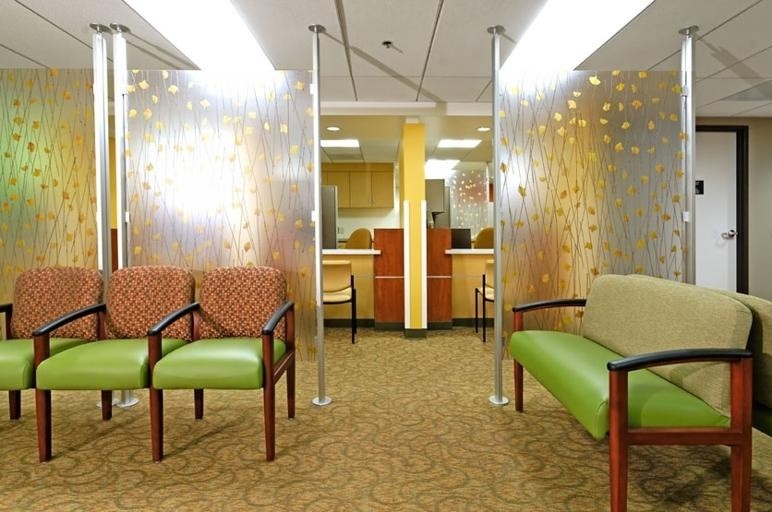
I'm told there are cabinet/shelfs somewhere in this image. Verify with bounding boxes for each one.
[350,171,394,208]
[374,227,404,323]
[425,177,444,213]
[427,228,453,323]
[321,171,350,208]
[432,185,451,228]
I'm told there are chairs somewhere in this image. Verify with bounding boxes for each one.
[320,260,356,344]
[475,259,495,342]
[474,225,494,249]
[346,227,372,249]
